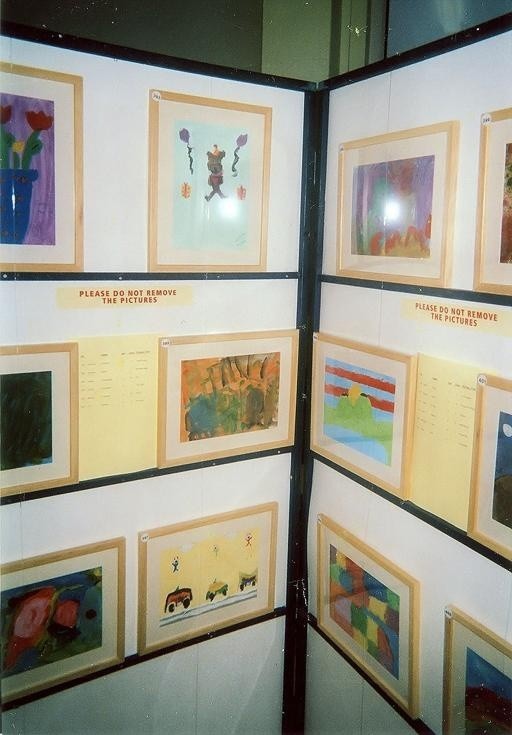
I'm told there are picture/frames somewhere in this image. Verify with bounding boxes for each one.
[337,119,453,288]
[316,515,419,719]
[470,108,512,300]
[1,341,80,496]
[1,538,127,704]
[146,87,271,274]
[309,331,415,501]
[1,62,85,274]
[466,374,512,561]
[157,330,299,469]
[138,501,280,656]
[441,603,512,735]
